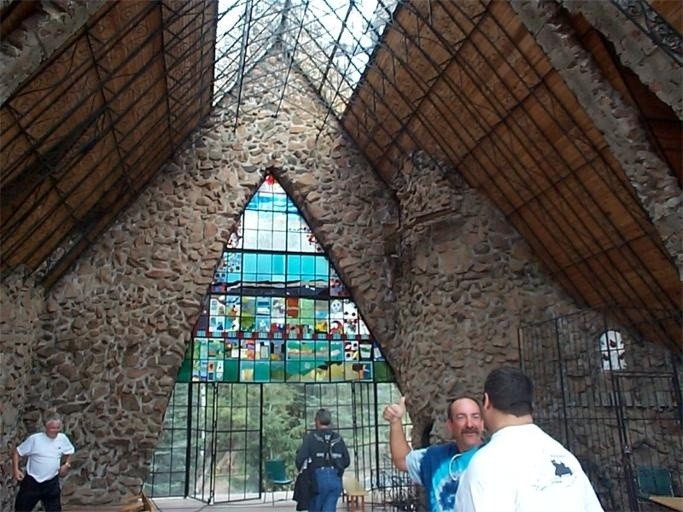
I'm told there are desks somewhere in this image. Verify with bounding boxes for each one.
[649,496,683,512]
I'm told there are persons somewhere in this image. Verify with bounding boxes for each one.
[295,408,350,511]
[452,366,606,512]
[13,412,75,511]
[382,396,485,512]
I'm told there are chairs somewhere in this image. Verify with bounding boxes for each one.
[264,459,293,508]
[637,466,675,512]
[341,471,368,512]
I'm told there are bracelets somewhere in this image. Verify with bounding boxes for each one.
[65,462,71,469]
